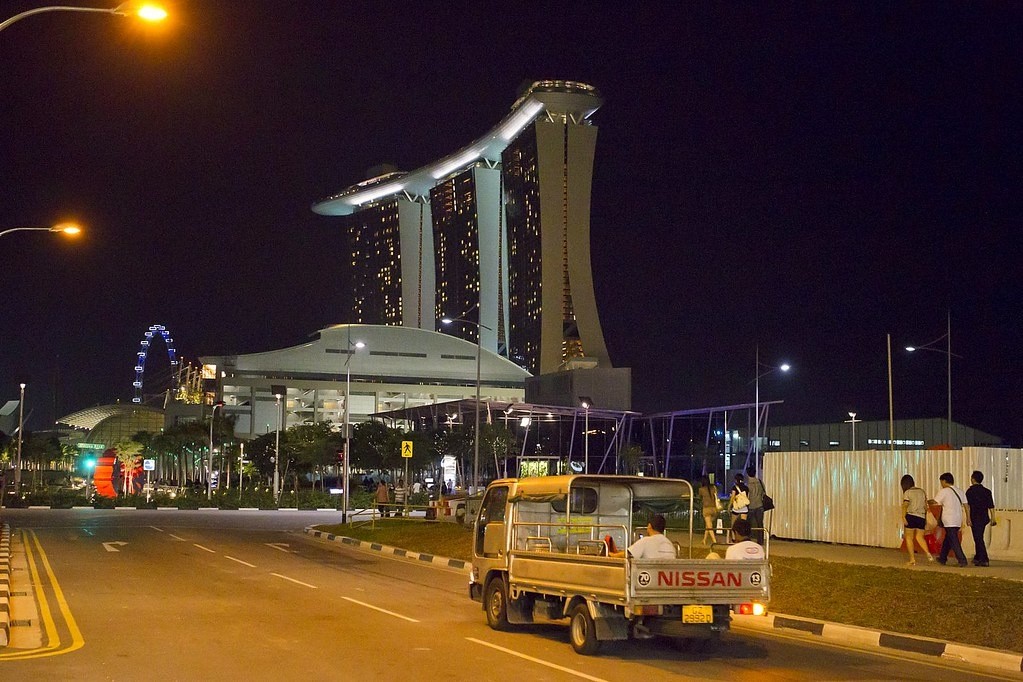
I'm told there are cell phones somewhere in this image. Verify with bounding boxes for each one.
[639,534,643,539]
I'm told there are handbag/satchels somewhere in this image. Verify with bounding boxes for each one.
[731,484,751,511]
[937,510,945,528]
[713,485,724,513]
[922,489,938,530]
[759,478,775,511]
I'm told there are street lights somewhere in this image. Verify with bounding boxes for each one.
[439,290,493,496]
[754,345,791,480]
[844,412,863,451]
[271,393,284,503]
[14,382,26,497]
[503,408,514,480]
[208,403,223,500]
[905,311,953,450]
[582,402,590,474]
[341,323,367,524]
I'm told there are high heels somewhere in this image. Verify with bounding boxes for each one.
[906,560,915,566]
[926,555,935,566]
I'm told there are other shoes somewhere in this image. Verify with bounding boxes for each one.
[935,557,946,565]
[958,561,968,567]
[971,558,989,567]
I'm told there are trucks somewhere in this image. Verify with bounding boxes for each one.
[456,474,774,655]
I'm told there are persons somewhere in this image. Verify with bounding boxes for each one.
[412,478,421,495]
[726,466,766,545]
[609,515,676,560]
[393,479,407,517]
[371,480,390,518]
[705,519,765,560]
[900,471,997,568]
[116,475,209,498]
[699,475,720,546]
[441,479,453,496]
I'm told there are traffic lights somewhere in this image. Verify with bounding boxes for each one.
[86,455,95,469]
